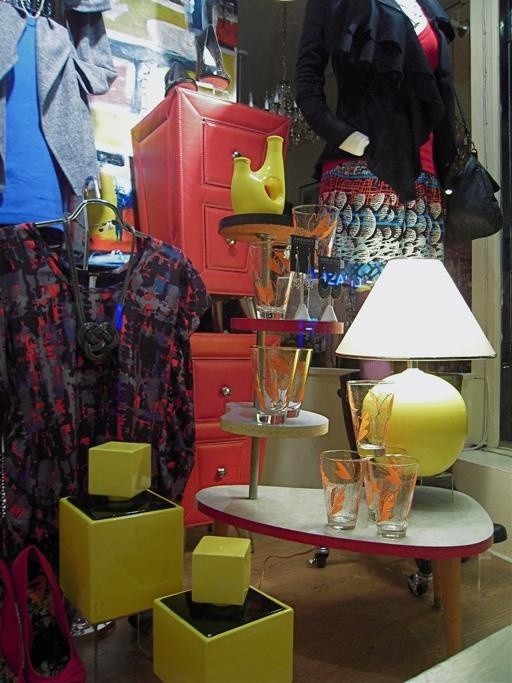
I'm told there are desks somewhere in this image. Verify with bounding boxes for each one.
[195,215,494,662]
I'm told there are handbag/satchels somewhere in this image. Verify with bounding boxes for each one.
[443,152,504,244]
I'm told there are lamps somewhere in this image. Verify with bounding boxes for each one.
[335,258,497,481]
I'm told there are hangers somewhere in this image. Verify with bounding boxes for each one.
[37,175,152,256]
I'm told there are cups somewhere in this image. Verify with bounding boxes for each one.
[320,380,420,538]
[247,205,341,425]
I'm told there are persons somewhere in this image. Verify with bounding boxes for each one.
[295,0,456,381]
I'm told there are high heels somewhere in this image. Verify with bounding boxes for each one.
[166,25,231,95]
[2,544,86,683]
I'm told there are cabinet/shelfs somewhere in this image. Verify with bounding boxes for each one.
[130,92,291,327]
[183,331,279,521]
